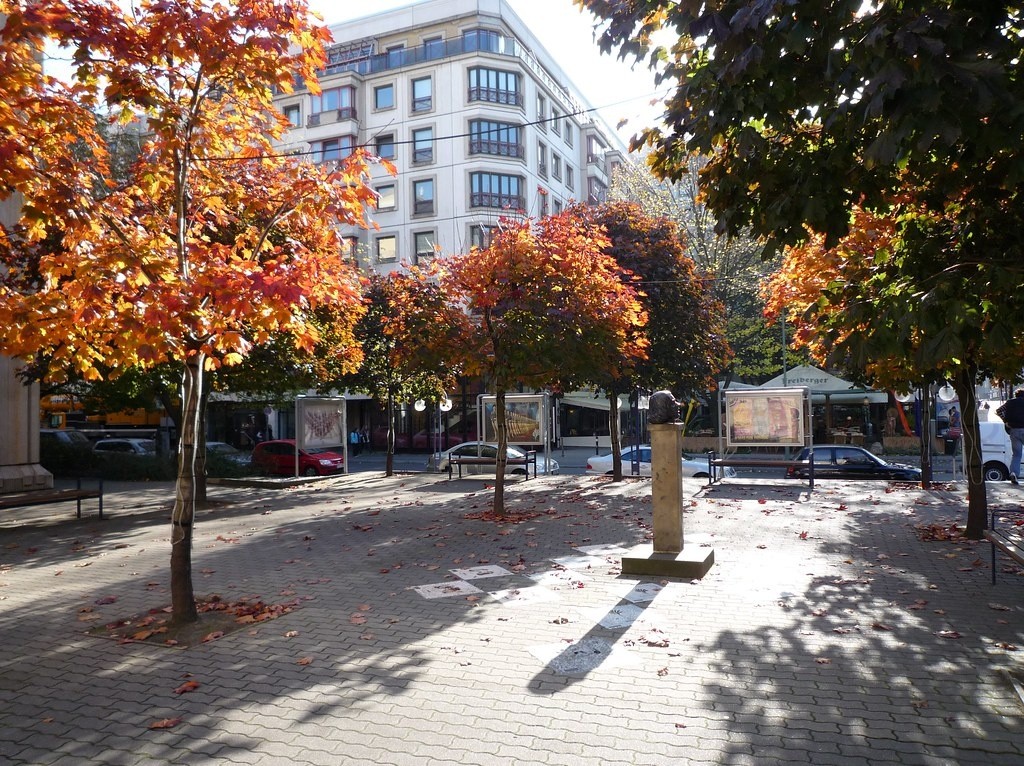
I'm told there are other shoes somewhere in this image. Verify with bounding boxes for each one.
[353,456,358,459]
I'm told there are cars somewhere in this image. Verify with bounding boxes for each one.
[206,441,251,477]
[371,426,409,451]
[91,438,159,478]
[584,443,737,479]
[250,438,345,476]
[427,440,560,476]
[411,428,462,452]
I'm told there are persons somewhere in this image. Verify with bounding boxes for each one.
[256,425,272,444]
[812,413,818,431]
[490,405,522,441]
[647,390,680,424]
[995,389,1024,484]
[984,402,990,411]
[350,425,374,458]
[949,406,960,427]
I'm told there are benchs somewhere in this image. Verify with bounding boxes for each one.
[982,508,1024,586]
[0,476,106,520]
[707,450,815,490]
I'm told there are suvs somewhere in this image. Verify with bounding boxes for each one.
[40,428,93,480]
[784,443,923,482]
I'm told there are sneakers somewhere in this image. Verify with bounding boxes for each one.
[1009,472,1018,485]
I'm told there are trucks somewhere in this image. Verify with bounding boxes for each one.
[978,420,1024,482]
